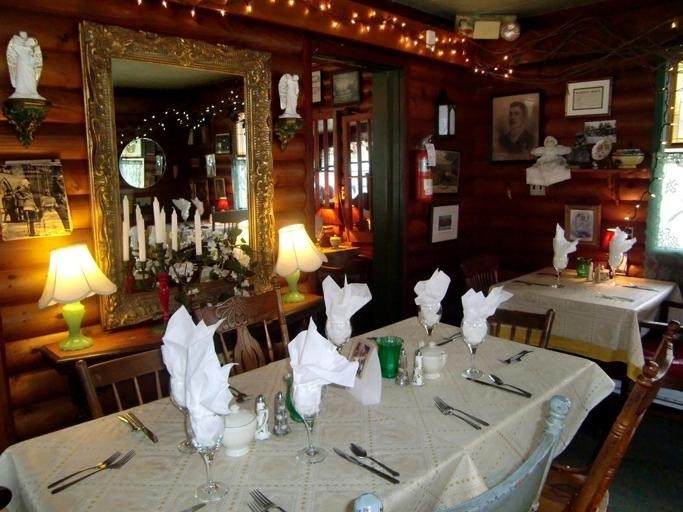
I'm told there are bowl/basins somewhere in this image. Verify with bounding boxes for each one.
[611,155,645,168]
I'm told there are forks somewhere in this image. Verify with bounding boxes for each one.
[497,350,527,364]
[511,350,535,362]
[247,487,286,512]
[47,448,122,489]
[246,499,267,512]
[50,449,136,494]
[432,395,489,426]
[433,398,481,430]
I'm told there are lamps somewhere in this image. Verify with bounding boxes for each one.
[38,242,118,351]
[276,223,328,304]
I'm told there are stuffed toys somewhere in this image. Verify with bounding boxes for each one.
[527,137,572,185]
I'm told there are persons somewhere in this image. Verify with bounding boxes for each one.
[1,188,16,222]
[498,100,537,154]
[16,179,40,237]
[41,190,65,235]
[13,186,29,222]
[48,164,70,233]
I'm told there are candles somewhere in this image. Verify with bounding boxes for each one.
[122,191,131,258]
[194,208,202,254]
[153,196,165,243]
[135,203,145,239]
[171,206,178,252]
[138,213,147,261]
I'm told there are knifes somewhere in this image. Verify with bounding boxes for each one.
[126,410,158,443]
[465,377,530,398]
[332,447,400,484]
[181,502,206,511]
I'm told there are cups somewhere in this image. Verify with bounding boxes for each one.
[575,257,592,278]
[288,383,328,465]
[169,379,197,454]
[417,304,442,337]
[325,321,353,355]
[551,253,568,288]
[376,335,402,378]
[184,412,229,503]
[460,320,491,379]
[607,252,624,287]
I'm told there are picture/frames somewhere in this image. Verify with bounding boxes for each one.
[430,150,459,193]
[564,205,602,246]
[488,88,541,163]
[432,205,459,241]
[331,70,361,106]
[311,70,322,103]
[0,160,72,241]
[583,119,618,143]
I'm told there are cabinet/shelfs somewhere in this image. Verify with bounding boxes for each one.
[41,291,324,432]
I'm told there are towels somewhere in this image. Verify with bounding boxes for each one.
[149,225,156,246]
[553,223,579,269]
[287,316,360,419]
[172,198,191,219]
[161,305,240,449]
[414,268,451,326]
[322,275,372,348]
[130,226,140,250]
[461,286,513,346]
[608,226,636,268]
[191,197,204,215]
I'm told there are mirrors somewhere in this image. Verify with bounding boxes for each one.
[79,22,276,331]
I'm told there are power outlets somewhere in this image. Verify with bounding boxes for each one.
[624,226,634,241]
[530,184,545,195]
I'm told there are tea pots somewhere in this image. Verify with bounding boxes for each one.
[415,338,448,380]
[220,404,268,457]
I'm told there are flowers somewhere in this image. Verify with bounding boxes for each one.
[132,223,253,303]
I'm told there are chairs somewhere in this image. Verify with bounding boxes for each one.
[459,250,500,295]
[531,320,681,512]
[608,301,683,411]
[75,348,166,417]
[190,286,291,375]
[313,256,376,290]
[483,309,556,350]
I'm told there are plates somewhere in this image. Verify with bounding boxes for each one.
[591,139,612,161]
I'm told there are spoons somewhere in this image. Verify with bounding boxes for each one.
[347,441,399,477]
[488,373,533,396]
[115,414,141,432]
